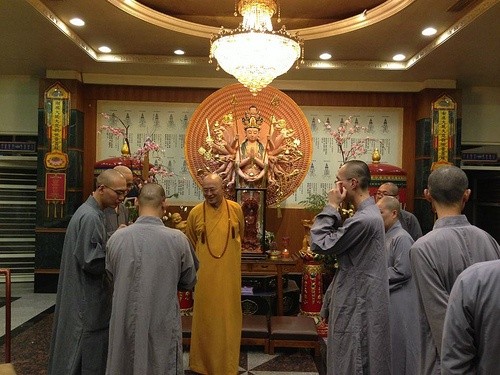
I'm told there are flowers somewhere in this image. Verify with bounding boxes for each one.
[97,111,188,222]
[317,116,384,164]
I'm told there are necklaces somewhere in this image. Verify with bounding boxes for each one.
[200,201,231,258]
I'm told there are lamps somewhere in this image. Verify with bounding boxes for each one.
[208,0,305,96]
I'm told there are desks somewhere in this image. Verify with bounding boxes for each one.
[242,258,307,331]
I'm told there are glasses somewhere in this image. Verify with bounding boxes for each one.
[98,184,127,196]
[334,178,358,185]
[375,192,397,197]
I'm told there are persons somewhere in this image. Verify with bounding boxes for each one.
[441,259,500,375]
[376,196,415,375]
[104,165,135,230]
[199,96,305,206]
[375,182,423,243]
[46,169,128,375]
[173,213,186,234]
[105,184,197,375]
[310,161,393,375]
[185,175,245,375]
[406,165,500,375]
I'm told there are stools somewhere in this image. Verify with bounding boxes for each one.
[181,316,192,347]
[242,315,321,358]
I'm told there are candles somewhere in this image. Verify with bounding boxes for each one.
[282,249,290,257]
